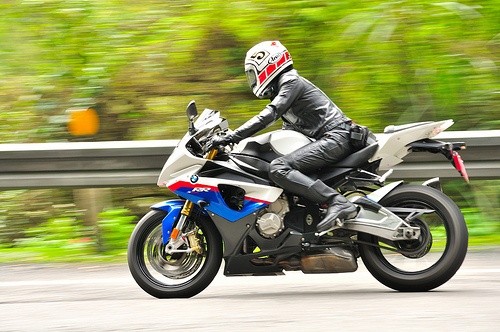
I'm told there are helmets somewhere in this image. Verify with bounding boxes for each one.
[245,40,294,99]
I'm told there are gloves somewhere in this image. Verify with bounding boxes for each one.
[211,133,231,150]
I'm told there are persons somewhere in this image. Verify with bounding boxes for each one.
[201,40,357,231]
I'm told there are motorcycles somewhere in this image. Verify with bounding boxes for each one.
[125,98,469,299]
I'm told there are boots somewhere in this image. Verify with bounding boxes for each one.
[304,179,356,232]
